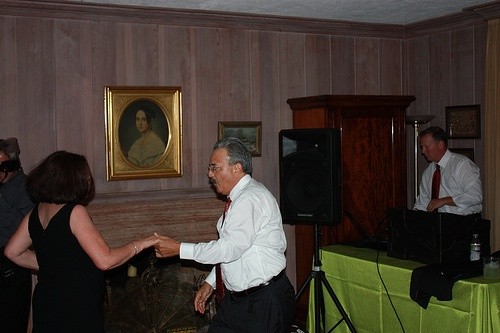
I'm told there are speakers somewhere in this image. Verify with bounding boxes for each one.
[278,127,341,225]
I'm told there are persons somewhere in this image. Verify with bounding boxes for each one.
[0,139,35,333]
[153,137,297,333]
[4,149,163,333]
[411,125,486,218]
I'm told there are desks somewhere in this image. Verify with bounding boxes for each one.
[305,242,500,333]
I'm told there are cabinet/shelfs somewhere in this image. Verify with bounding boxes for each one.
[286,93,416,321]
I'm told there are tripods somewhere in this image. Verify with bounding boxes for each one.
[294,224,358,333]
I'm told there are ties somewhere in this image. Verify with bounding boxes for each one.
[431,164,440,212]
[221,198,232,228]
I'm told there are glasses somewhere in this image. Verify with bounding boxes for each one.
[208,162,236,173]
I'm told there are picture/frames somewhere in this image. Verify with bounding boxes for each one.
[103,85,184,182]
[218,120,262,158]
[445,104,481,140]
[447,147,474,163]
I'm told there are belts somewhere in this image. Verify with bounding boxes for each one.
[227,267,285,301]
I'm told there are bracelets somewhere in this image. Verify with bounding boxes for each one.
[130,242,138,255]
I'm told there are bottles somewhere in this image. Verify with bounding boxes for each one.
[469,233,480,261]
